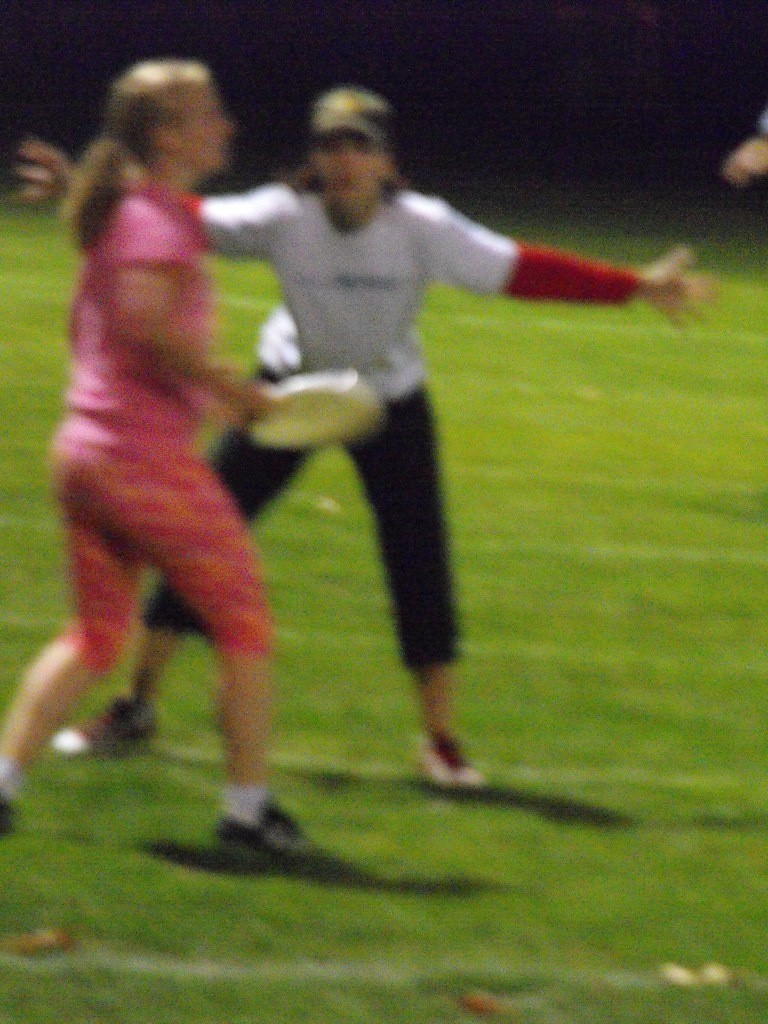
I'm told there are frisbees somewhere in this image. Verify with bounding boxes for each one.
[248,365,388,454]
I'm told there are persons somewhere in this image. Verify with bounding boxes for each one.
[0,54,328,858]
[15,82,715,793]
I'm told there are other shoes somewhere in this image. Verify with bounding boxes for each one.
[213,806,320,869]
[417,734,484,793]
[55,699,157,757]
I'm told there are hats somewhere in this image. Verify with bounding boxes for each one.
[314,91,396,148]
